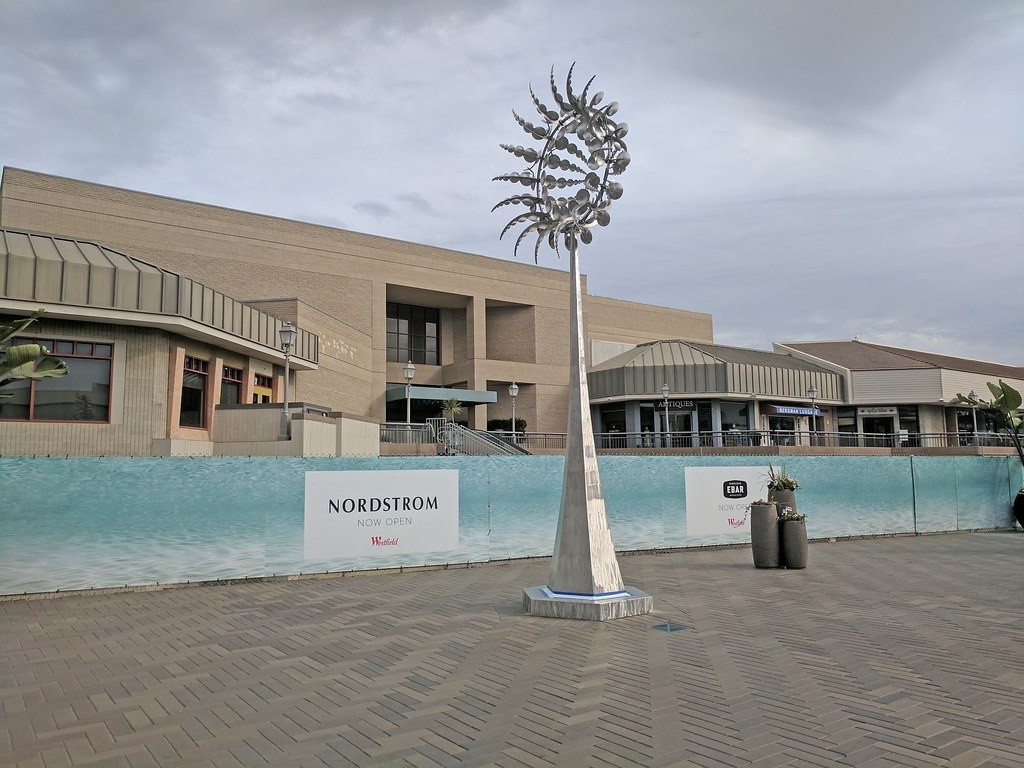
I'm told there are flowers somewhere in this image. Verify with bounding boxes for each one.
[777,505,808,522]
[741,495,777,522]
[756,461,802,492]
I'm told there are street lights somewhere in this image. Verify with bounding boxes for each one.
[402,360,416,430]
[508,381,520,445]
[278,320,298,442]
[661,383,672,447]
[807,385,818,446]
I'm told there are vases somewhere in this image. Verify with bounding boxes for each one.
[768,488,796,517]
[751,505,780,569]
[781,522,809,569]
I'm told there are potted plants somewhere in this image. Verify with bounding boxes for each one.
[948,379,1024,529]
